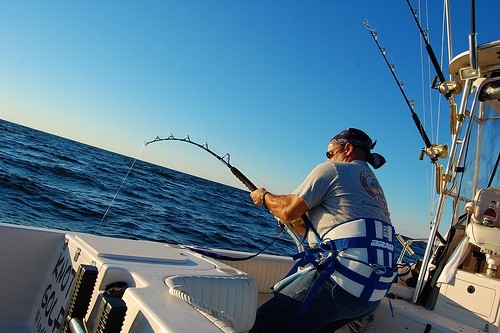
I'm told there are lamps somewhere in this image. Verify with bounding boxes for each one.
[459,65,481,80]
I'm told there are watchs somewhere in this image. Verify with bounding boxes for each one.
[259,191,269,207]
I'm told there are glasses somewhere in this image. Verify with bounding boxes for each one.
[326,144,345,159]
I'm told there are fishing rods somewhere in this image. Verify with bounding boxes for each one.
[405,1,462,138]
[360,18,451,198]
[93,134,312,267]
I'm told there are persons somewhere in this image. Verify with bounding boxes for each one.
[250,128,399,332]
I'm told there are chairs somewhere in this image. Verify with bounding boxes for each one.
[464,186,500,280]
